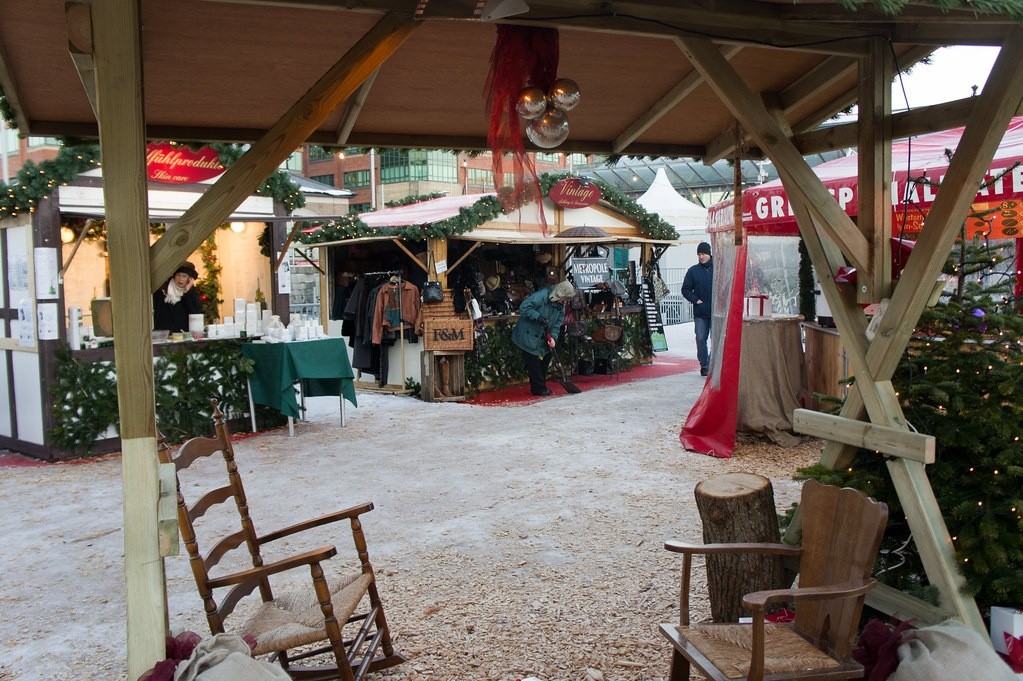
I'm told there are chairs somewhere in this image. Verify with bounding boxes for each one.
[160,396,409,681]
[659,479,890,681]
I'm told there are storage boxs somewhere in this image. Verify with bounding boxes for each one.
[422,317,474,352]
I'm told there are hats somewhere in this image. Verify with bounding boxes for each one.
[173,261,198,280]
[549,281,577,302]
[611,281,629,298]
[487,275,500,290]
[697,242,711,255]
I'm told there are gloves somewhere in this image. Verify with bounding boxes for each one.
[538,316,549,327]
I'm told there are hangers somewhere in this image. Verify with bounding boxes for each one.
[365,271,401,283]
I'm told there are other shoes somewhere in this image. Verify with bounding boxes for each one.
[533,388,552,396]
[701,366,707,376]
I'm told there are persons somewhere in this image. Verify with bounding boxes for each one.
[511,281,576,396]
[152,262,203,336]
[680,242,728,376]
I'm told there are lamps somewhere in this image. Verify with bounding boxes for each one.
[60,222,75,243]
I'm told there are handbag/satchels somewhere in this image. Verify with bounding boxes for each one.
[423,251,443,303]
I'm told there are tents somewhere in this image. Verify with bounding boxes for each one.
[634,167,708,231]
[679,85,1023,458]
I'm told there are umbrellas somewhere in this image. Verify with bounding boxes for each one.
[554,223,612,258]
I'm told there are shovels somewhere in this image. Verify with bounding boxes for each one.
[541,318,583,393]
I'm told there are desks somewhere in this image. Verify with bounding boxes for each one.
[243,337,359,437]
[801,320,855,413]
[735,313,807,445]
